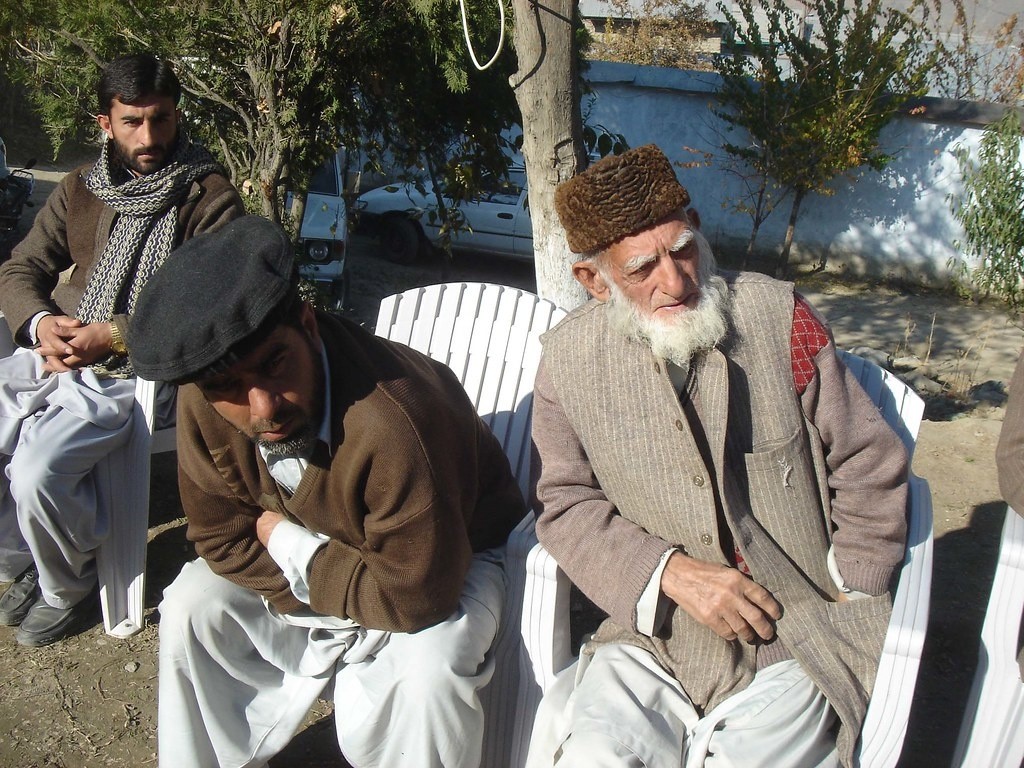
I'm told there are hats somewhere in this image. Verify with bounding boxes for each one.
[553,143,690,257]
[126,211,301,385]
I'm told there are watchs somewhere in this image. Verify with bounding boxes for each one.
[109,321,129,356]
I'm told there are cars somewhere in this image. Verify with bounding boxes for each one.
[350,154,605,266]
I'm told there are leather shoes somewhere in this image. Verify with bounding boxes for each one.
[0,560,40,627]
[15,589,104,648]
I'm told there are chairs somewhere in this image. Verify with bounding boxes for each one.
[510,345,936,768]
[0,312,179,638]
[950,504,1023,768]
[373,279,567,768]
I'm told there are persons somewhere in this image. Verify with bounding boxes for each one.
[530,143,914,768]
[996,347,1024,685]
[1,51,249,652]
[128,218,528,768]
